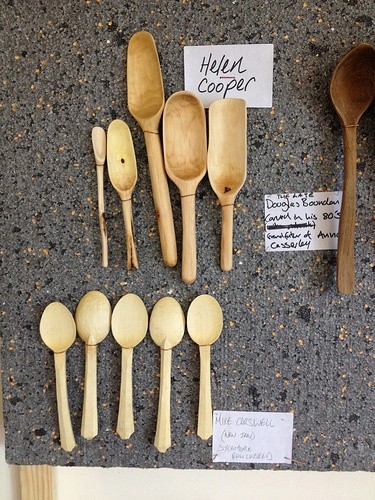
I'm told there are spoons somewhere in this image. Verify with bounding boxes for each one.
[109,293,148,439]
[107,119,139,272]
[205,98,247,272]
[185,293,224,440]
[149,295,187,455]
[91,124,109,269]
[37,300,76,453]
[162,91,208,285]
[328,41,375,294]
[126,30,178,267]
[74,290,113,440]
